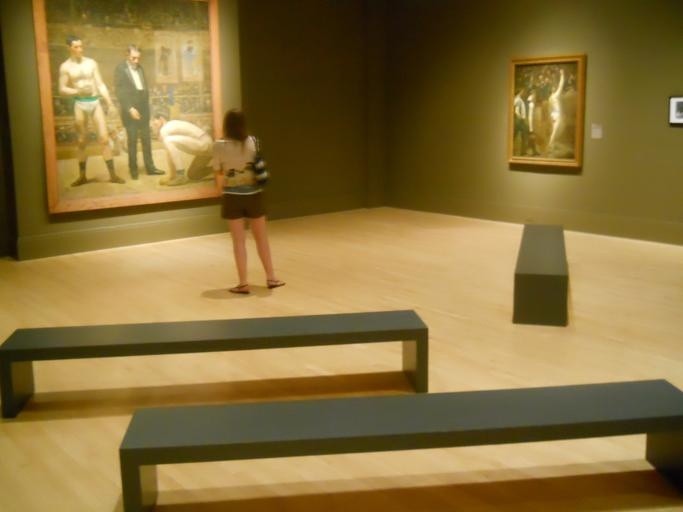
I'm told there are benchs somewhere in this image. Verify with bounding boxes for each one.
[1,306,430,420]
[511,222,572,328]
[117,377,683,512]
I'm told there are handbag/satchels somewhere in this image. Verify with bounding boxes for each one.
[253,157,268,185]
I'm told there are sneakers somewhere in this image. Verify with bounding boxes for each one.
[71,177,88,186]
[110,175,125,183]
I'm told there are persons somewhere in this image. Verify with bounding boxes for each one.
[213,108,286,294]
[57,35,127,187]
[513,65,576,158]
[112,44,166,179]
[148,111,214,184]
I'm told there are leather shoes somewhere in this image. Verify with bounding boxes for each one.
[132,168,137,179]
[147,169,164,175]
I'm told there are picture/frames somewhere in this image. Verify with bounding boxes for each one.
[507,52,589,173]
[30,0,226,219]
[666,94,683,127]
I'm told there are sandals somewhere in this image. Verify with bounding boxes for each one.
[267,278,285,289]
[229,284,249,294]
